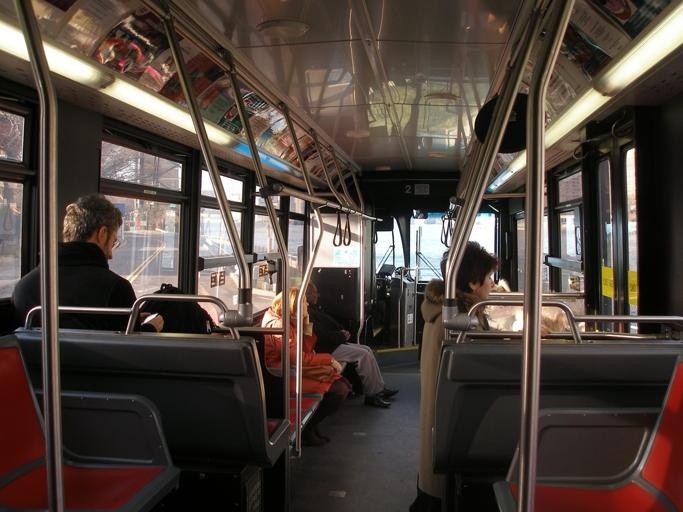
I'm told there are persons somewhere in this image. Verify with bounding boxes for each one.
[410,240,497,511]
[261,287,351,447]
[8,192,165,334]
[296,281,400,409]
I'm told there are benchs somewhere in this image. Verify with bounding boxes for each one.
[436,299,681,481]
[12,293,290,509]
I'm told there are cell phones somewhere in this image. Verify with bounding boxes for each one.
[141,313,159,325]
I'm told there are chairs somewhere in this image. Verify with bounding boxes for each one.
[491,353,681,510]
[0,337,181,509]
[209,307,347,461]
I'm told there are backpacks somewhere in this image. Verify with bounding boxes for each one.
[135,284,216,334]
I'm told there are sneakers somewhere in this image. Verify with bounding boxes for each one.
[301,428,330,445]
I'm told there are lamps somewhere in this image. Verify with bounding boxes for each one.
[255,19,308,39]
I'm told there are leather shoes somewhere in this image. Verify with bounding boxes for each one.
[365,395,391,407]
[380,388,398,396]
